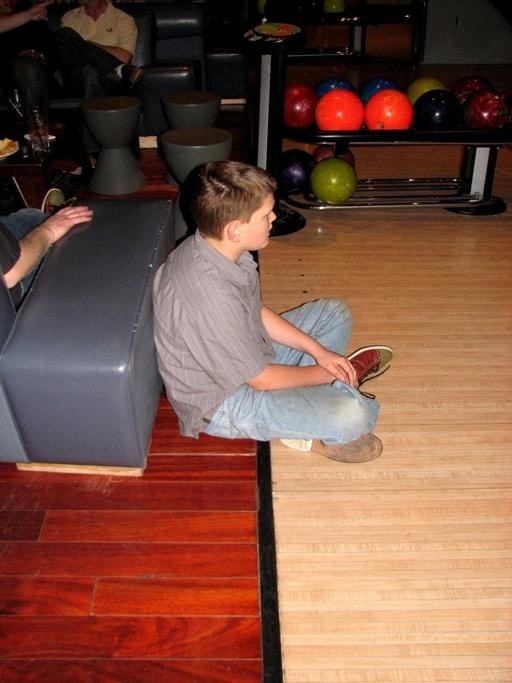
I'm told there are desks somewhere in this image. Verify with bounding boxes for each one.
[281,128,511,215]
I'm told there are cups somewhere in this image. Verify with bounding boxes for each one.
[28,106,50,152]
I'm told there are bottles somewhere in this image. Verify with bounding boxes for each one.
[7,88,25,121]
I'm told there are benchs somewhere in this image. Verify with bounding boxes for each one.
[1,195,178,477]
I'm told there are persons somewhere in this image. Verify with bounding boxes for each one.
[151,157,394,464]
[0,201,95,308]
[0,0,56,35]
[51,0,147,170]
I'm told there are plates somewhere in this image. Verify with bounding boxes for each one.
[0,141,19,160]
[23,134,57,140]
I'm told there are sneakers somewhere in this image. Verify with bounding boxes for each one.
[281,433,382,463]
[347,345,393,384]
[42,188,65,216]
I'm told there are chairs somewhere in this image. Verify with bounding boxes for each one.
[35,2,247,153]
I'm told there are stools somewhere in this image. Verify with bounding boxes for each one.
[161,129,233,233]
[79,96,147,195]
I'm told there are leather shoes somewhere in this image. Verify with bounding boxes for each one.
[121,64,143,87]
